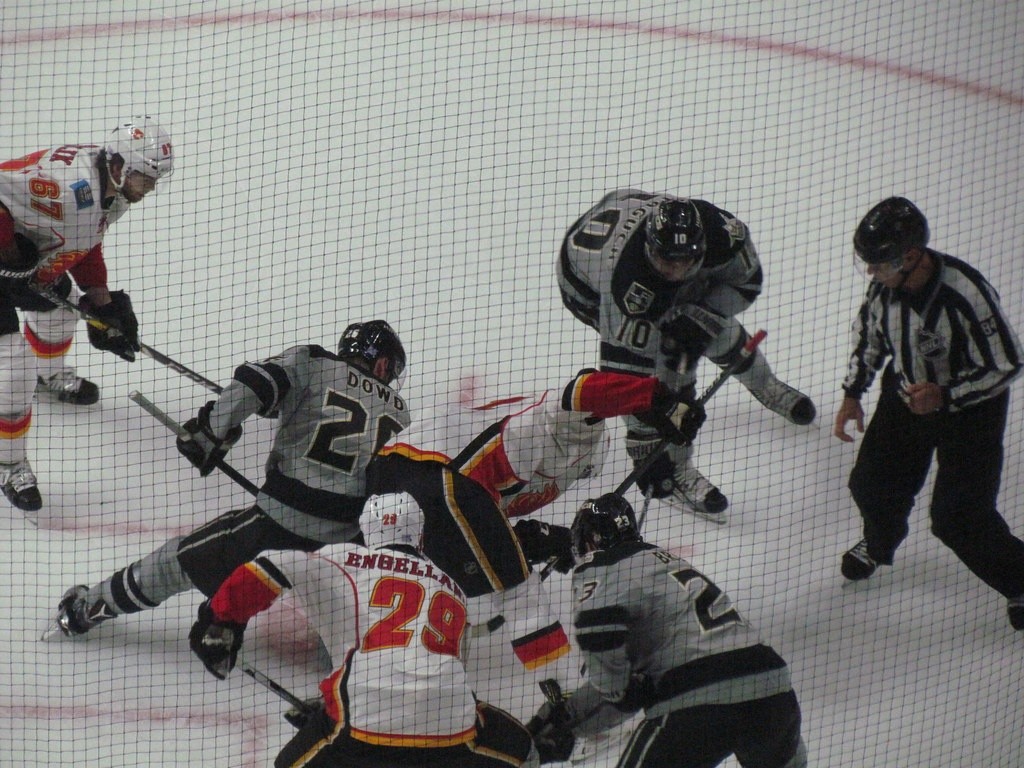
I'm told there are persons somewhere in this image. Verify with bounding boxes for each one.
[41,321,808,768]
[556,188,820,525]
[834,196,1024,632]
[0,116,173,512]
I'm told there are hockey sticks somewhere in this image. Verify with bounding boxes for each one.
[125,388,260,506]
[19,285,277,415]
[236,657,314,716]
[457,324,774,641]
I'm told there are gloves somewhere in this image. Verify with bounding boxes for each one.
[598,678,647,714]
[515,519,575,574]
[79,290,142,362]
[175,400,244,478]
[657,298,729,371]
[624,430,675,499]
[0,232,72,312]
[528,702,577,764]
[187,599,247,680]
[633,380,682,426]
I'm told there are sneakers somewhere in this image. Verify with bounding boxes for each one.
[41,585,119,642]
[1006,594,1024,631]
[566,715,631,761]
[32,369,104,411]
[750,373,822,430]
[0,461,43,527]
[661,463,729,525]
[840,537,881,581]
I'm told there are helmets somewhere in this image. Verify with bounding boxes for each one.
[571,493,640,564]
[646,196,708,260]
[359,491,425,553]
[852,196,928,263]
[104,117,175,180]
[337,319,406,379]
